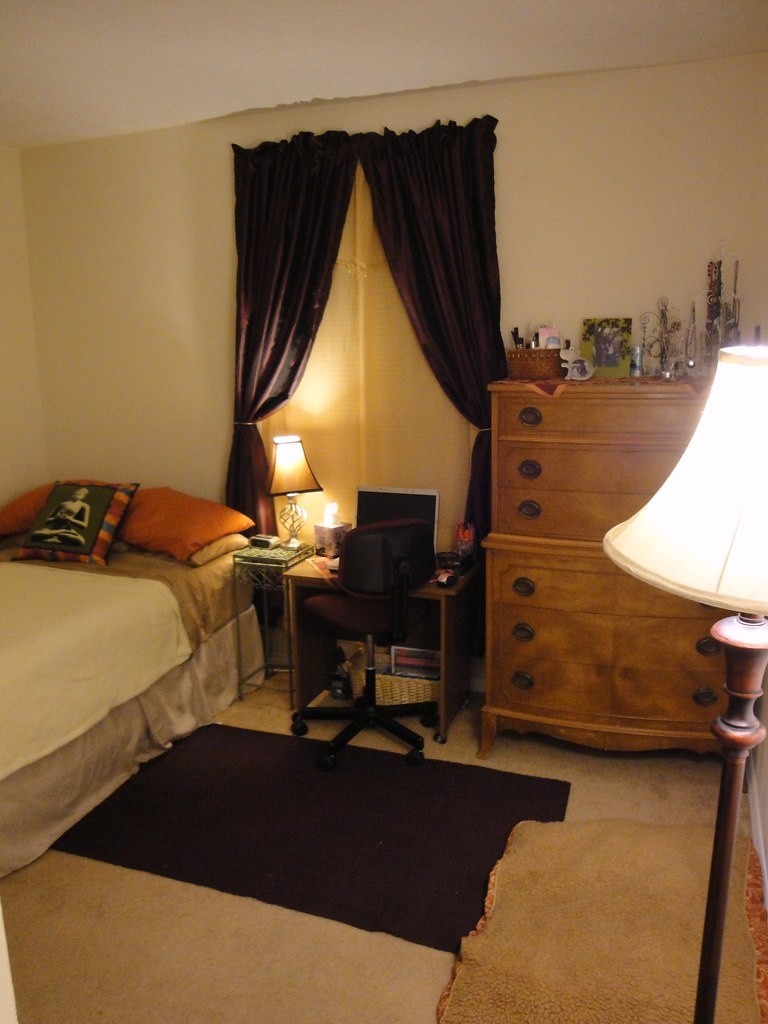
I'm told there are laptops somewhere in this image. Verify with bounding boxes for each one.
[328,487,440,573]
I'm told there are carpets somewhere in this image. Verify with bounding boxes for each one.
[47,723,573,954]
[435,818,768,1024]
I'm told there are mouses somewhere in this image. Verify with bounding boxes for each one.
[436,572,457,587]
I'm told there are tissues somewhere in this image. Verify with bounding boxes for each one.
[314,502,352,559]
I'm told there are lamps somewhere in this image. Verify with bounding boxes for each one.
[604,344,768,1024]
[265,435,323,551]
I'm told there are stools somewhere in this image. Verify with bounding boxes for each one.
[232,543,316,708]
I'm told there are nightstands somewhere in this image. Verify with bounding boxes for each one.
[285,555,478,743]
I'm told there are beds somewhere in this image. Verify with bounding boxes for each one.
[0,480,269,879]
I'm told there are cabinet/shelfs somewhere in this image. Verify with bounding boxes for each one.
[477,377,755,794]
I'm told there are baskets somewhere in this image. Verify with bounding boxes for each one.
[349,649,440,706]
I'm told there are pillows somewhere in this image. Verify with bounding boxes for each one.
[0,481,113,534]
[9,481,141,567]
[119,487,255,562]
[188,533,250,567]
